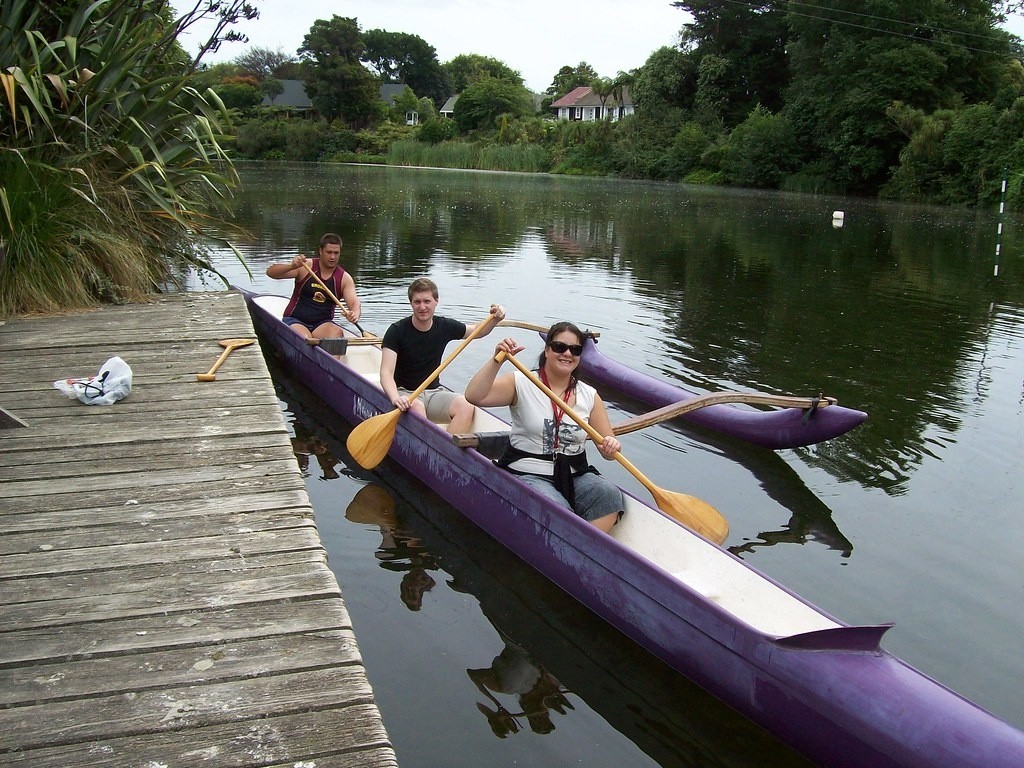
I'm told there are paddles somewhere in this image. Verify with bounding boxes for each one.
[293,257,383,350]
[494,348,731,548]
[345,303,505,470]
[343,484,398,527]
[195,338,256,381]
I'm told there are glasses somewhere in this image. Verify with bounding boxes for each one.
[547,341,583,356]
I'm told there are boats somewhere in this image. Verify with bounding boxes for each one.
[228,282,1023,768]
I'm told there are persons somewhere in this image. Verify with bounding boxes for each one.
[464,321,626,534]
[266,233,362,360]
[380,279,507,437]
